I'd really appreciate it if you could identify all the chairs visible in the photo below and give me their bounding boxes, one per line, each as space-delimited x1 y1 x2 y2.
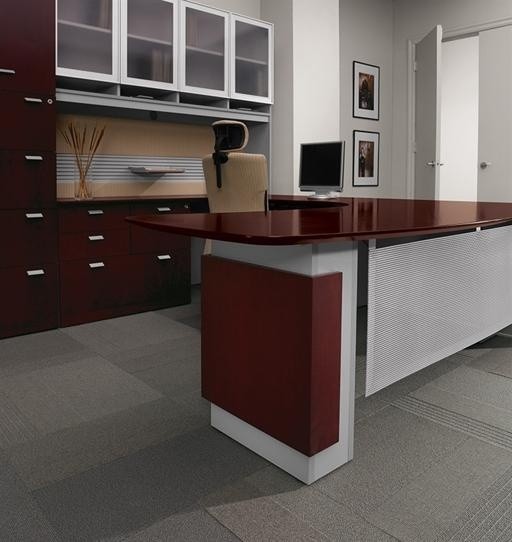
202 119 272 255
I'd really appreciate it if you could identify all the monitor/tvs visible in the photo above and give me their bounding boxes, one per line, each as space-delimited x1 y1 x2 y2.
299 140 346 199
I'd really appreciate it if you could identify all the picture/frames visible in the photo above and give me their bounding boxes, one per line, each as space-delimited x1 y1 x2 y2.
352 130 379 186
352 60 380 120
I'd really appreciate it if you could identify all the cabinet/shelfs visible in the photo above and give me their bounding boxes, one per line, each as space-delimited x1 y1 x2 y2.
0 0 58 338
177 0 275 105
55 0 177 90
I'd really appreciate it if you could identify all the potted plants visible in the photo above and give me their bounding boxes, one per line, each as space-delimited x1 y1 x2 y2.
56 120 105 200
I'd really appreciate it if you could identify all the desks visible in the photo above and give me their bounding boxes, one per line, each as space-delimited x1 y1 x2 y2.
59 191 512 487
